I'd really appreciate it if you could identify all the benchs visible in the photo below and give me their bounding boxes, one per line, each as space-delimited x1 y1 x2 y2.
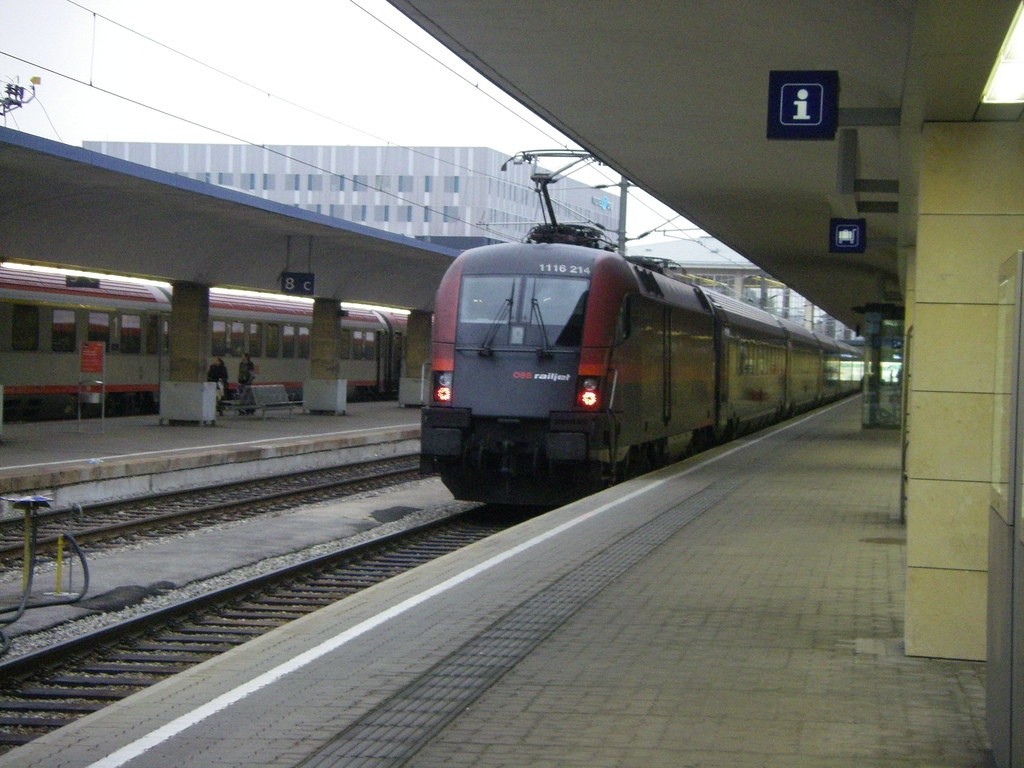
220 385 258 419
250 385 308 423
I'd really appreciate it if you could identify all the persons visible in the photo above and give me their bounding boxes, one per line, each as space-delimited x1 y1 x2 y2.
238 350 257 415
208 358 233 418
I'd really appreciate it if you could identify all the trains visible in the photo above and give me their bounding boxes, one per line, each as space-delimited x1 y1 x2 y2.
1 267 412 427
419 220 902 507
